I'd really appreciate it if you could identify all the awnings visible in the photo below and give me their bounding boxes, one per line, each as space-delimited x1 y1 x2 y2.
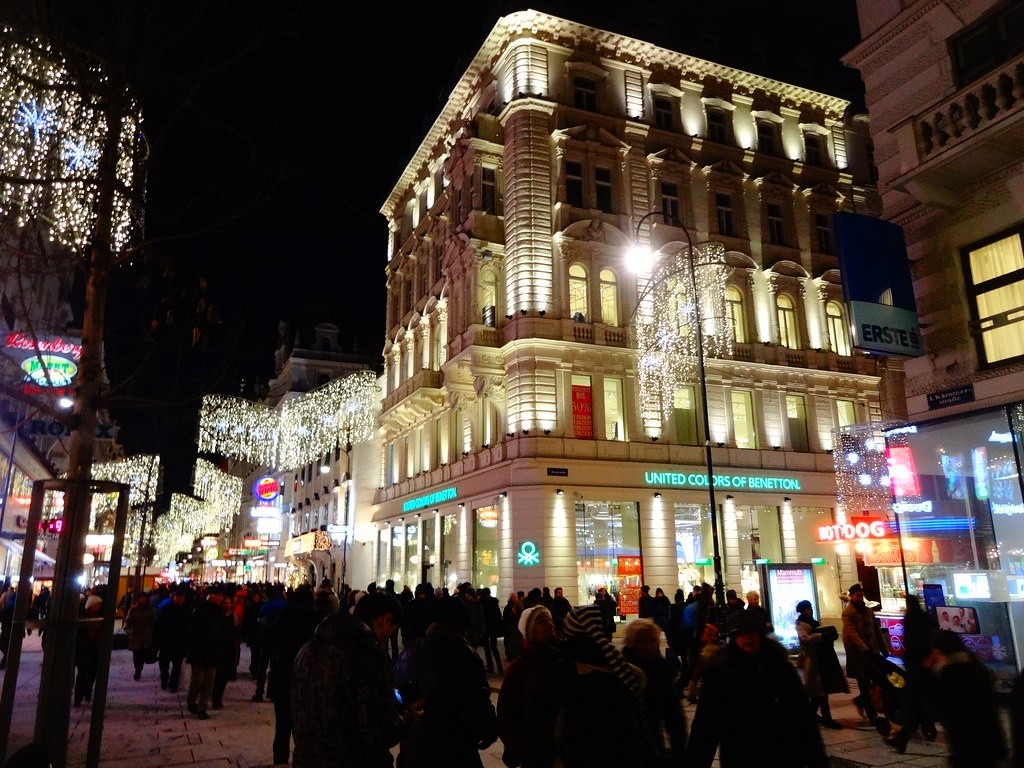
1 538 57 569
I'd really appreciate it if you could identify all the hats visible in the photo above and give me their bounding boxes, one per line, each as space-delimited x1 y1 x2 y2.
562 606 658 712
624 619 659 649
796 600 811 612
849 584 862 595
518 604 552 641
725 609 773 636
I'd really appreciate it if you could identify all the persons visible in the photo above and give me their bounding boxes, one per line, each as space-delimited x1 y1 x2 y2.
637 584 772 703
495 588 688 768
941 608 976 633
0 584 50 670
843 584 933 754
74 582 106 707
273 580 503 768
117 579 285 720
935 629 1024 768
687 616 827 768
796 600 850 728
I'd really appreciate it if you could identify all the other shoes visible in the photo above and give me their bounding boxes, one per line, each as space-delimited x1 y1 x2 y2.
187 703 198 714
484 663 494 672
823 721 842 729
86 695 92 701
854 698 868 719
197 710 208 720
883 737 906 754
213 701 223 710
167 683 177 693
161 679 168 690
687 695 699 704
74 702 81 707
249 695 263 702
134 671 141 681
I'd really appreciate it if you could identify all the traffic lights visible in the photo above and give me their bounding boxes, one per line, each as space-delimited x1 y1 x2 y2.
48 518 62 534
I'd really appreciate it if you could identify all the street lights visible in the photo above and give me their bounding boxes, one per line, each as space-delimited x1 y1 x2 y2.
319 445 351 590
624 211 725 604
174 520 243 586
1 364 76 540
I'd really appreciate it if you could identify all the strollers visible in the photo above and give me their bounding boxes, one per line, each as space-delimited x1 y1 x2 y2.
859 652 939 746
